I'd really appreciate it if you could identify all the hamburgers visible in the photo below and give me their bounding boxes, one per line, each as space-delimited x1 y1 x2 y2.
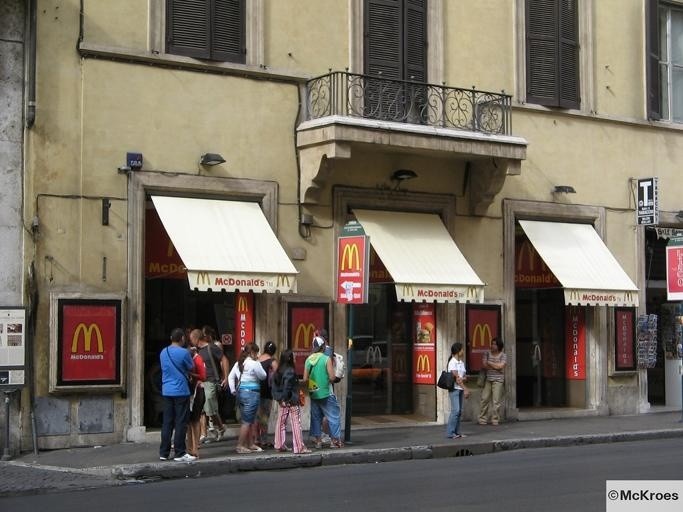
419 329 430 342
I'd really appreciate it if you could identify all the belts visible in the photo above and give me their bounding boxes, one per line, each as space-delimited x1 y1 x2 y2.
238 387 262 394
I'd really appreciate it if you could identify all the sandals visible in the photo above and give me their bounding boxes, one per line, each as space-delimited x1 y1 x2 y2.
234 445 252 454
248 445 264 452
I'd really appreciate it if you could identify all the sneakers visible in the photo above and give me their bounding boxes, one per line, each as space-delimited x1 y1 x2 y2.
255 440 273 449
303 448 312 454
276 445 292 452
198 415 226 446
173 452 199 462
160 453 172 461
312 432 345 449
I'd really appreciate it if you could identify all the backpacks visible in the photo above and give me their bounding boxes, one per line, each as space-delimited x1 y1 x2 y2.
332 352 346 383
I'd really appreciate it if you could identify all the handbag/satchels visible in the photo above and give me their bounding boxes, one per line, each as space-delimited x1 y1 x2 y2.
188 373 196 395
234 396 241 422
437 371 455 390
298 389 304 406
216 377 226 393
476 367 487 388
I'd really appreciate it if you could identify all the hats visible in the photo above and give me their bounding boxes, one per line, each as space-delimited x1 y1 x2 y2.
311 335 325 352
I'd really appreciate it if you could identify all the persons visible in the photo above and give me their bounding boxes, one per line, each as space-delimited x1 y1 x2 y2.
270 349 312 454
301 336 345 449
253 339 277 450
227 341 268 455
202 325 224 433
444 342 469 439
157 328 197 464
475 336 508 425
189 330 230 448
184 344 207 461
312 416 331 449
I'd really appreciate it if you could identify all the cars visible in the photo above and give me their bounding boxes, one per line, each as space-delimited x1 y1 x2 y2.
351 358 387 384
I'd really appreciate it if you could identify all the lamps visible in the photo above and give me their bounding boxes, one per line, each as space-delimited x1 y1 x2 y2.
392 168 419 181
200 153 226 167
553 185 576 194
